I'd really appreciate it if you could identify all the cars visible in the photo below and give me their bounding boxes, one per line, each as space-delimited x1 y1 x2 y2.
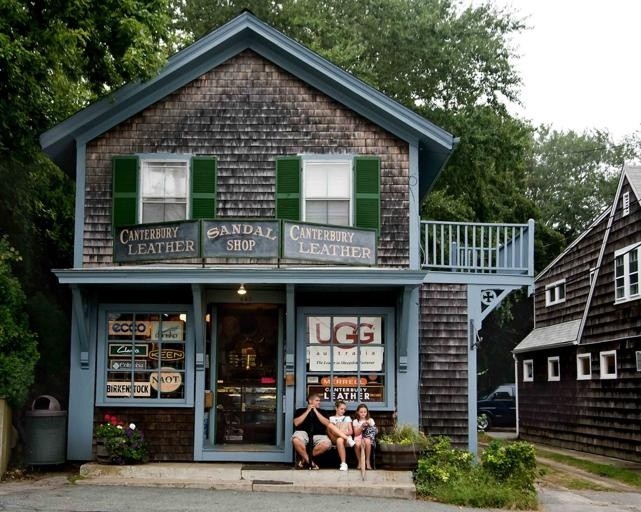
242 412 278 439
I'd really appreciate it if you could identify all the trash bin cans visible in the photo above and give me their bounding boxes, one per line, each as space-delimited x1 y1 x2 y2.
24 395 67 471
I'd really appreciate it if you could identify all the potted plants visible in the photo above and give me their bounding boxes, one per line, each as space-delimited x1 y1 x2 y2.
375 421 430 472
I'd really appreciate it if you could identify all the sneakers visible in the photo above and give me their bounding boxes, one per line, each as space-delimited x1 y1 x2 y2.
339 463 348 471
346 437 356 447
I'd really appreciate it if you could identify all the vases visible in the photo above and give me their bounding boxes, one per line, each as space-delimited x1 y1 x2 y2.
96 437 112 464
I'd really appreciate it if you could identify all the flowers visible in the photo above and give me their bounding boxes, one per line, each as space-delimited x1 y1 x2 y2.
94 412 151 466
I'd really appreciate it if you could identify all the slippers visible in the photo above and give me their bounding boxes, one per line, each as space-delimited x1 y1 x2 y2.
356 464 373 470
295 460 319 471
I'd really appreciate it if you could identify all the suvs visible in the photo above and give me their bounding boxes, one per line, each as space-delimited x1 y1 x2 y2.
477 383 517 432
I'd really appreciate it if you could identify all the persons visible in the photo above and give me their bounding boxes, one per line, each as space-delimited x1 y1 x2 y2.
353 403 377 470
326 401 355 471
292 394 332 469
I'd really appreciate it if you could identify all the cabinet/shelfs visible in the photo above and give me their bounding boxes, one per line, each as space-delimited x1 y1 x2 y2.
217 380 277 443
